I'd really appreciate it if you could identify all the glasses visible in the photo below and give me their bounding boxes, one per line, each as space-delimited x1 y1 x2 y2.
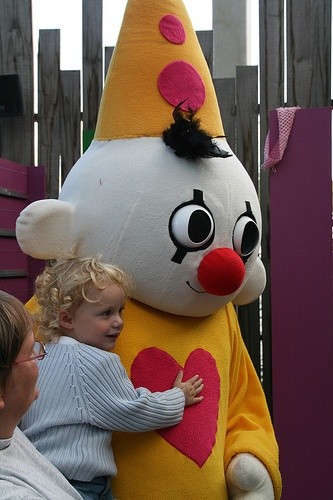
11 340 48 366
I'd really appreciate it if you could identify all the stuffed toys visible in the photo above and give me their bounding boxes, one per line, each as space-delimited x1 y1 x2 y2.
16 0 281 500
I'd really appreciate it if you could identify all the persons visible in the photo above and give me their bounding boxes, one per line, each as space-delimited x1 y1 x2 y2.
0 290 84 500
16 252 204 500
